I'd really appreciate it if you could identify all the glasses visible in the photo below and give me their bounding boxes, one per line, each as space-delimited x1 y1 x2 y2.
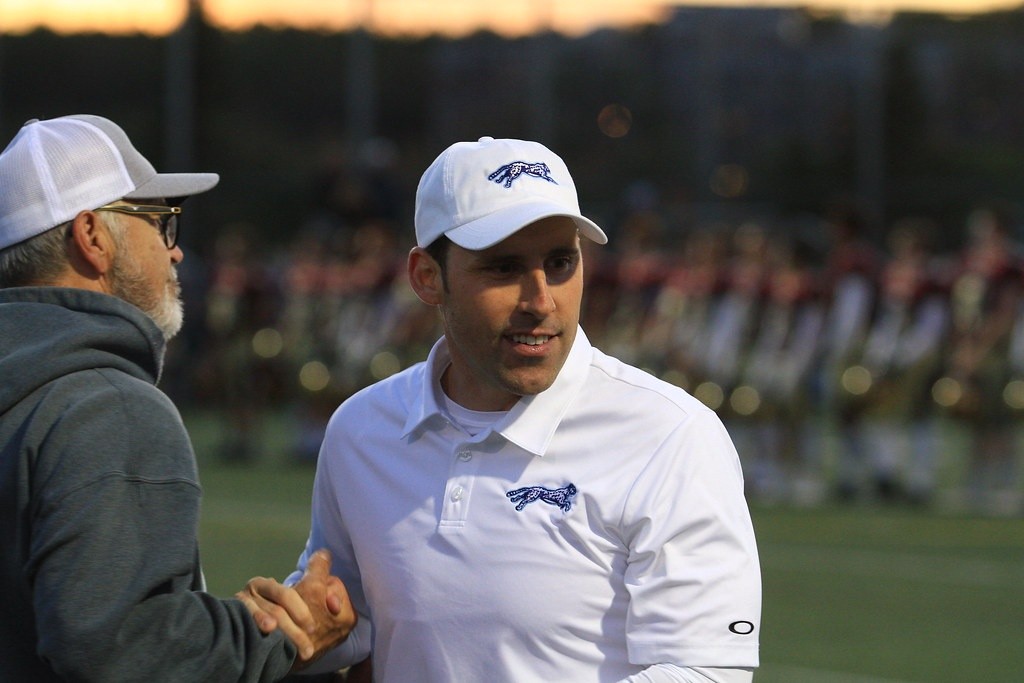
93 204 180 250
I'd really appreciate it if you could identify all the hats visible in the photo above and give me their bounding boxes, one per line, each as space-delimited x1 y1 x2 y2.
414 136 608 251
0 115 219 250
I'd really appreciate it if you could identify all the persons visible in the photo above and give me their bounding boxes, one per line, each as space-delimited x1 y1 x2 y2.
209 208 1024 511
232 135 764 683
0 110 356 682
